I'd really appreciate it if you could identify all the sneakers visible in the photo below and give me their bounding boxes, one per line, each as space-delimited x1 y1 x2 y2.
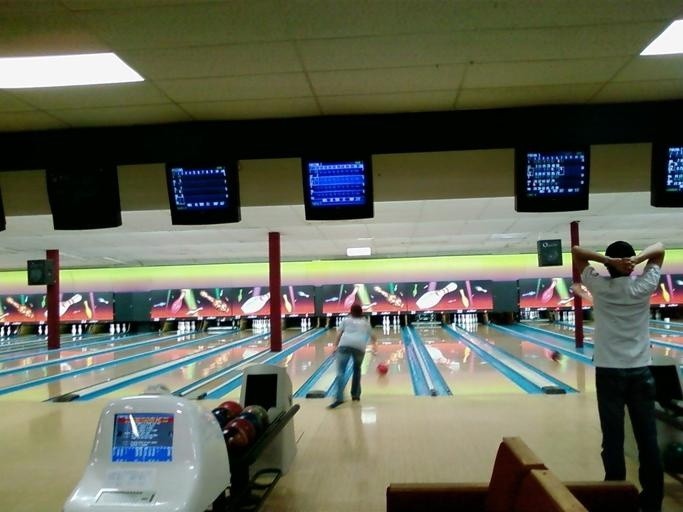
324 396 362 409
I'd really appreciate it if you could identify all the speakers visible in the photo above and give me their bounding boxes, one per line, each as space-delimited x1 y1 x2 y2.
27 260 54 285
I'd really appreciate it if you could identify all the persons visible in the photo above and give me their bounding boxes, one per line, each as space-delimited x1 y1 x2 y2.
567 239 667 512
325 304 379 410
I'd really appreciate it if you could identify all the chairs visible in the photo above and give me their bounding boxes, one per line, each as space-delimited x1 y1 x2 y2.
386 439 640 512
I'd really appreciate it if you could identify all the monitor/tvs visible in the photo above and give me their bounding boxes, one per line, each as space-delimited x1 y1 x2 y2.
245 373 276 408
647 365 682 399
302 151 375 221
43 162 124 231
514 141 591 213
112 412 172 465
165 161 242 225
650 137 683 208
